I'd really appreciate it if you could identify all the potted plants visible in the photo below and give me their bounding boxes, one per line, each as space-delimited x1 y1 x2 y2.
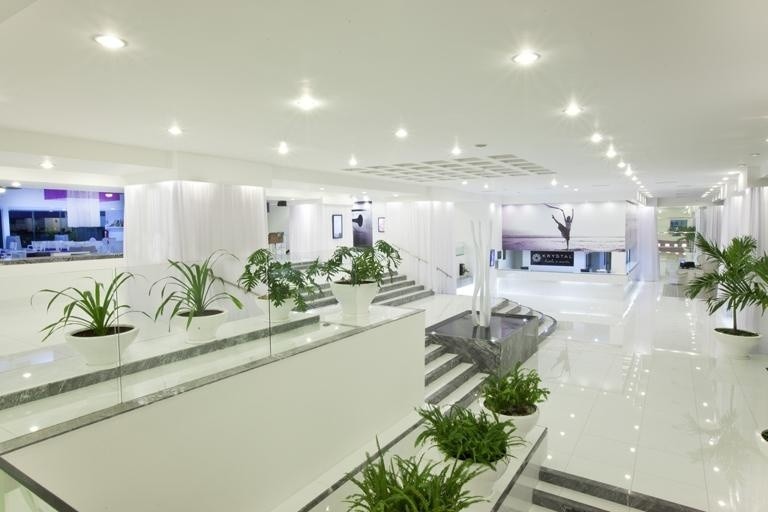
338 434 493 511
30 272 157 366
149 248 246 344
411 402 528 499
684 232 768 359
477 361 552 448
236 248 325 323
319 239 403 318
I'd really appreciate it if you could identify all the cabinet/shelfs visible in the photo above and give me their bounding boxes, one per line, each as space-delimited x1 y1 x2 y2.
383 399 551 512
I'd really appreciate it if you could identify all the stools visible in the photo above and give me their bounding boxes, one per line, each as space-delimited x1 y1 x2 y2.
665 263 689 285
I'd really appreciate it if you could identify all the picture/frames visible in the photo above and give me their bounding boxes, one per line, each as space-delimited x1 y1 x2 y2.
332 214 343 238
498 251 501 259
490 249 495 267
378 217 384 232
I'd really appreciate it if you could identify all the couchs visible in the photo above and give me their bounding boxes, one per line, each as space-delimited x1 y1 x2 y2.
696 252 721 285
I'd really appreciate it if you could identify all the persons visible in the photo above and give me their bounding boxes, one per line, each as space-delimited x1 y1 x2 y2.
552 208 574 249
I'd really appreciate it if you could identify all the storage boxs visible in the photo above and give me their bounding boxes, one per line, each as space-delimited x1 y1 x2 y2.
268 233 286 256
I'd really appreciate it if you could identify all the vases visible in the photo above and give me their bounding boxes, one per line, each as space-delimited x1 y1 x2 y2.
758 428 768 446
